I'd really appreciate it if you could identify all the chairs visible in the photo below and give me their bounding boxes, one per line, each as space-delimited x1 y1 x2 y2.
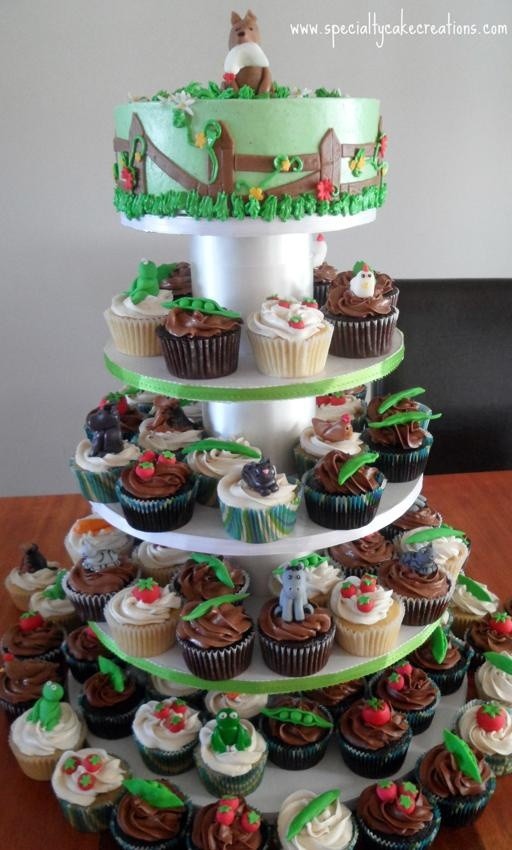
363 273 509 476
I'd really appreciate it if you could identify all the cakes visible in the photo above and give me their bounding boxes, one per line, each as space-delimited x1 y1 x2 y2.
0 9 512 850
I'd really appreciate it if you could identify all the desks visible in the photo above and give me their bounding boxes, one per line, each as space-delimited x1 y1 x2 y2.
1 463 511 847
